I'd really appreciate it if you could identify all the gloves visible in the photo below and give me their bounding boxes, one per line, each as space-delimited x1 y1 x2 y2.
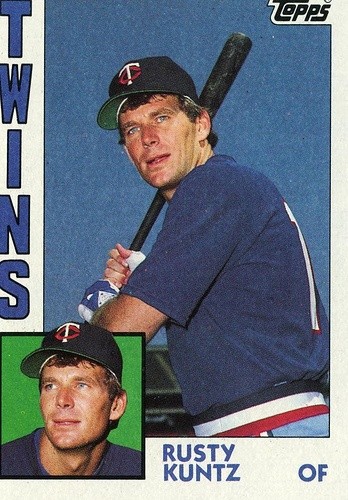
78 279 121 324
125 250 146 273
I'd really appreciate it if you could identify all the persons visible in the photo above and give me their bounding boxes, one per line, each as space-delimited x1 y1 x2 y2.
0 320 142 476
77 56 329 438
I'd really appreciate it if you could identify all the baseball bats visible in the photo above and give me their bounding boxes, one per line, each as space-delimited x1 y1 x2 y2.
129 32 253 252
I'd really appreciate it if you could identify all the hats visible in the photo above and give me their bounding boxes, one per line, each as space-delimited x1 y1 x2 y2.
19 321 123 385
97 55 200 131
